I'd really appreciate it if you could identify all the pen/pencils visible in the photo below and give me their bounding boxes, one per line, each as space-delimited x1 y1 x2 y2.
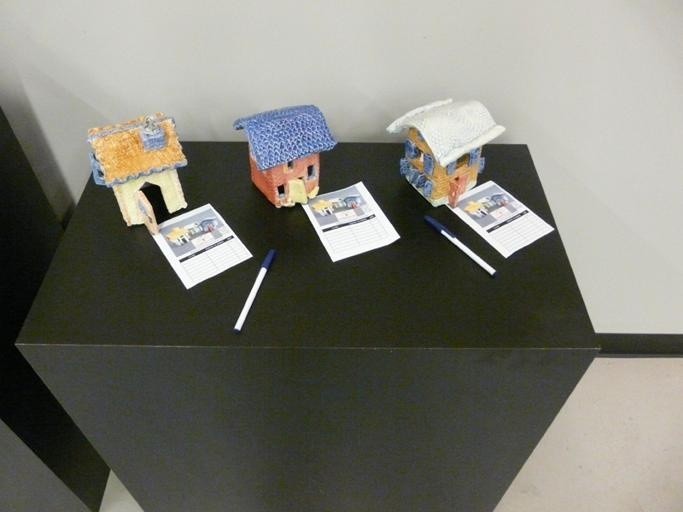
234 249 275 334
424 216 497 277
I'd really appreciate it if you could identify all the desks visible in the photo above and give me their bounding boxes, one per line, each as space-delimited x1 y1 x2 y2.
15 141 602 512
